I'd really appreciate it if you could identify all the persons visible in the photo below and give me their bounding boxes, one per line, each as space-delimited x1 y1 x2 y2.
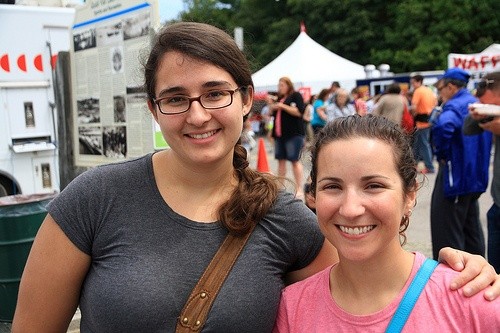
8 20 500 333
243 74 441 200
462 71 500 275
270 113 500 333
428 68 494 263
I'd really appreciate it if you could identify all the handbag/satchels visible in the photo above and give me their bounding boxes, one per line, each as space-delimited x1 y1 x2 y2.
402 111 414 134
415 113 430 123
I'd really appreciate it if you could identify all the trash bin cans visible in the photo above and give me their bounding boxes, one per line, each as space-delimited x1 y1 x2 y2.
0 190 58 323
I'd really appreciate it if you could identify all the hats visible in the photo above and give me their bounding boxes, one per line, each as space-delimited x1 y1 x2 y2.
434 67 472 88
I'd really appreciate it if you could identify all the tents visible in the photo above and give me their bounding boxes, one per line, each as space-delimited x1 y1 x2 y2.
251 22 394 96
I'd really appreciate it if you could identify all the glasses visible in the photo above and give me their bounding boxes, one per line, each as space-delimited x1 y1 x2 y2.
152 85 244 116
480 79 495 89
437 84 446 91
